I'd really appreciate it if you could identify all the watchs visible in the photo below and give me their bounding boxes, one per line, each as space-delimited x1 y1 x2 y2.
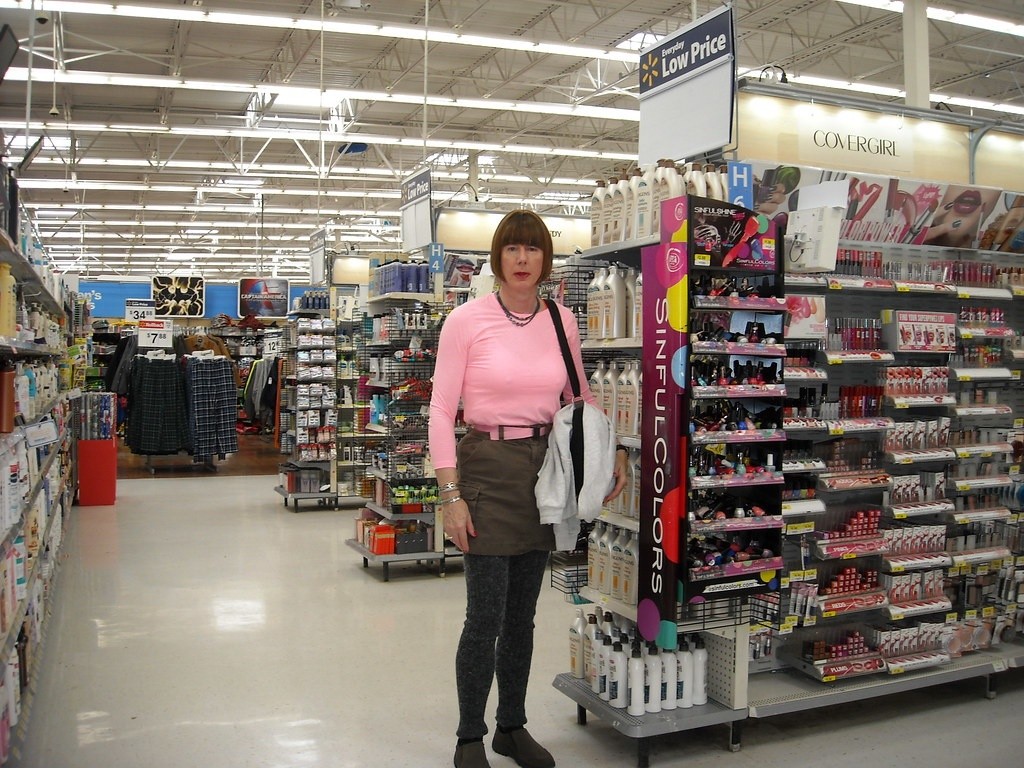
616 444 630 460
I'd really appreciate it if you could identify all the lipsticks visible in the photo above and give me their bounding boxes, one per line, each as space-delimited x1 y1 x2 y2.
840 176 1022 252
802 247 1024 662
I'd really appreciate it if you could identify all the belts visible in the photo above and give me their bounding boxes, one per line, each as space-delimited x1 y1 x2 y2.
473 424 546 441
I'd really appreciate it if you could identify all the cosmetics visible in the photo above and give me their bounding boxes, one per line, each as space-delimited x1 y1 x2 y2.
689 273 830 685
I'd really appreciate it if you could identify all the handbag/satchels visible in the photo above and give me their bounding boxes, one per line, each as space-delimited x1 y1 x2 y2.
569 406 586 497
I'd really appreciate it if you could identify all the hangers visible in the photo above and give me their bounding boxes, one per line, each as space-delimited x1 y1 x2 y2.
135 317 232 371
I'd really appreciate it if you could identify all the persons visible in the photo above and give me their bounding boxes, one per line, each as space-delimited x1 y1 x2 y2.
428 209 630 768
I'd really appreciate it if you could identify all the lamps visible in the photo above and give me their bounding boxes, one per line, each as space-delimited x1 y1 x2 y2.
0 1 910 277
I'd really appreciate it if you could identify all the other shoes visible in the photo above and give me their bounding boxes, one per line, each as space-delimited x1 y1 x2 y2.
491 727 556 768
454 742 491 768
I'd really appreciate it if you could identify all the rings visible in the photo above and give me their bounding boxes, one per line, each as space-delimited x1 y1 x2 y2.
446 536 453 541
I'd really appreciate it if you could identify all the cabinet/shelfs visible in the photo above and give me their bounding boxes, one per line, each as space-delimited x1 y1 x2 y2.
555 160 1024 765
209 311 283 444
1 151 96 765
346 241 613 578
88 320 135 437
272 275 375 513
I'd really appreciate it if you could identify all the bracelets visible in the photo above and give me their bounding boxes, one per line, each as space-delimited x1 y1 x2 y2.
440 495 460 507
437 481 460 495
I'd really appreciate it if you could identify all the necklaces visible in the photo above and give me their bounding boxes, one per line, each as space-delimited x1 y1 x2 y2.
496 290 541 327
